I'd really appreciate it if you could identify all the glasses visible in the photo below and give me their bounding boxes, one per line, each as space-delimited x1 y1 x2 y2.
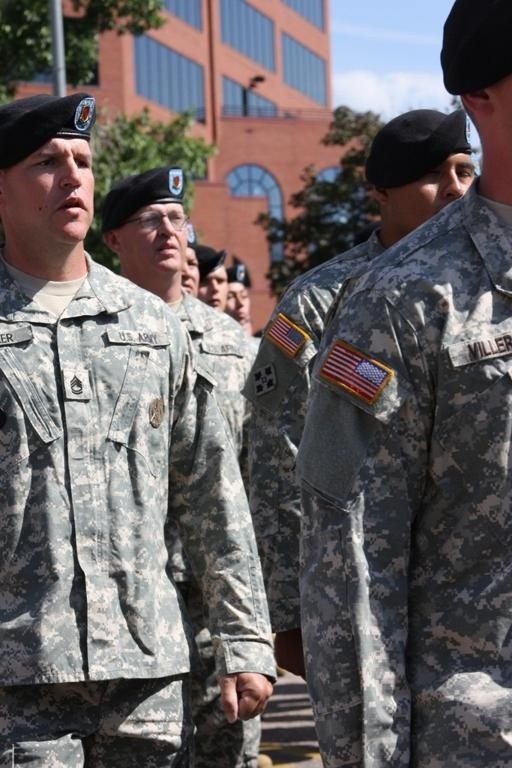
124 211 189 229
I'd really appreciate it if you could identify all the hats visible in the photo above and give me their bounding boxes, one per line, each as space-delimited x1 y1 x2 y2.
102 167 186 231
441 0 512 95
0 92 95 168
365 109 472 186
197 245 250 286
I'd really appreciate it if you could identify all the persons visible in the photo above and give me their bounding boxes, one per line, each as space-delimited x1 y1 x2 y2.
0 89 286 768
94 166 274 768
246 109 479 684
297 0 512 768
